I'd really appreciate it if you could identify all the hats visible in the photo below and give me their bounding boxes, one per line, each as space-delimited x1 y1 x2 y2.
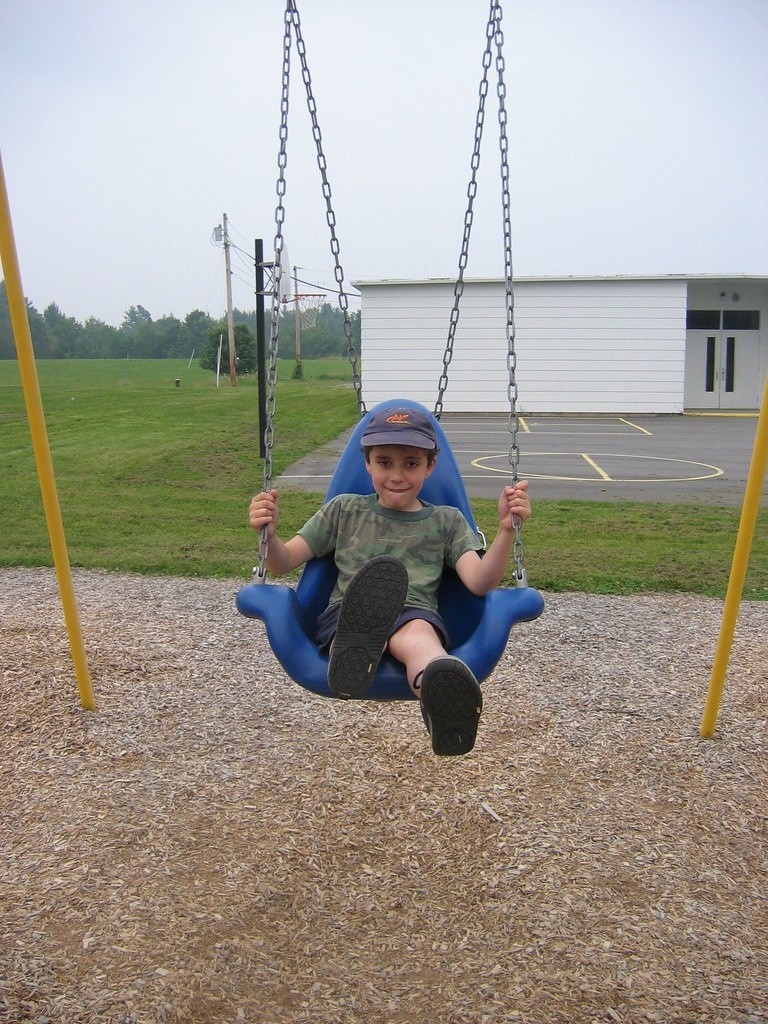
360 407 440 450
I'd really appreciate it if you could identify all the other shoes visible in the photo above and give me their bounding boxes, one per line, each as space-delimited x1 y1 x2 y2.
327 556 408 702
413 655 483 756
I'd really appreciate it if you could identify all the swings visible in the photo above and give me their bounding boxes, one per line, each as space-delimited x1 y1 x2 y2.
232 0 548 705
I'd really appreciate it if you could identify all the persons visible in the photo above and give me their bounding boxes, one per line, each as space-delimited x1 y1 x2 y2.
250 407 532 756
